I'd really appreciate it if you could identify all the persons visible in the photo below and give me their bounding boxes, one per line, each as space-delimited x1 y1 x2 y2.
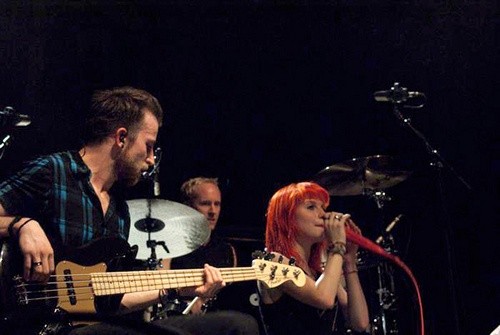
256 182 370 335
156 177 237 270
0 87 261 335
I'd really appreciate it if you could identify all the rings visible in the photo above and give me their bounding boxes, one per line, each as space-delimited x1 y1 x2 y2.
33 261 42 266
335 216 340 219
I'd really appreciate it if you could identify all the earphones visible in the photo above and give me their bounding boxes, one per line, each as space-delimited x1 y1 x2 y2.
120 132 124 143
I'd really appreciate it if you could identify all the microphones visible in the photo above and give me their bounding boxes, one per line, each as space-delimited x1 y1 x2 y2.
344 227 393 258
373 82 428 103
374 214 402 245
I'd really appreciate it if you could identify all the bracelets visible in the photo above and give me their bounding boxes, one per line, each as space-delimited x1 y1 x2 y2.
159 287 180 303
326 240 348 257
8 216 33 238
344 270 359 275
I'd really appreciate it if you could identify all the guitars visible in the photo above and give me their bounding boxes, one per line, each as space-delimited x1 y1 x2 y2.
0 231 308 335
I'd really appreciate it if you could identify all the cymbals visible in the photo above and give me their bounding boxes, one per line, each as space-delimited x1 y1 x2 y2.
310 154 417 196
123 199 211 260
